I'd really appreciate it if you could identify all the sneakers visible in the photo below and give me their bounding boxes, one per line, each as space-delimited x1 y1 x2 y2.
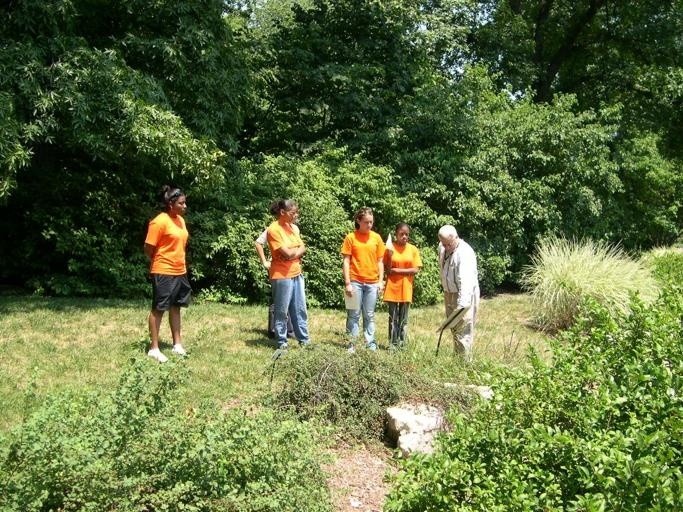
148 349 168 363
173 344 184 353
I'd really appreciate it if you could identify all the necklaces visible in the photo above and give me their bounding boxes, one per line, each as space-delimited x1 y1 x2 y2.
443 238 461 262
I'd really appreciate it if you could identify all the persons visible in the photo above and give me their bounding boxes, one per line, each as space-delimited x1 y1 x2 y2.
383 220 423 346
266 199 314 349
144 186 190 364
254 199 308 340
340 205 383 351
435 226 481 366
